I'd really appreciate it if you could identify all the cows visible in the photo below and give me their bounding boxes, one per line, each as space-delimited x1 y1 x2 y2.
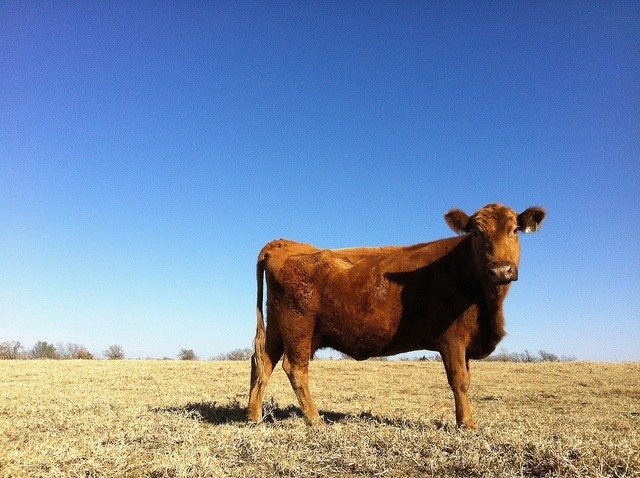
246 200 549 433
